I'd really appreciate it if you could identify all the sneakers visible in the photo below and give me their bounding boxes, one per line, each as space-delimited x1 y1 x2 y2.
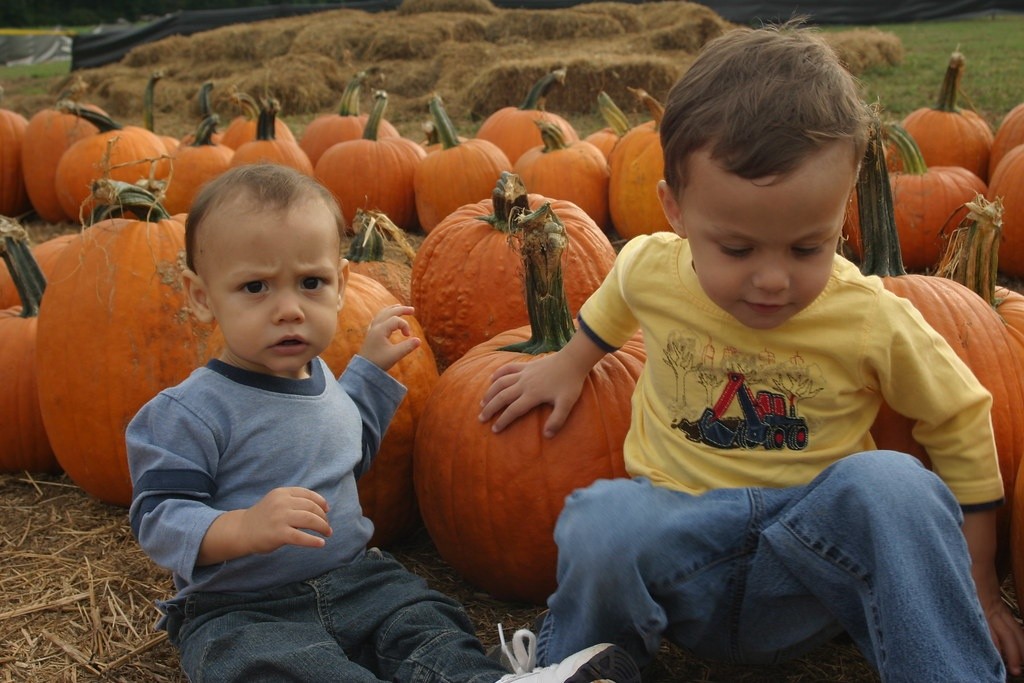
496 642 641 683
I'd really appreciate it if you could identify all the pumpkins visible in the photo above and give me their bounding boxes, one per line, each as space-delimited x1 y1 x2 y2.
836 50 1024 619
0 71 669 601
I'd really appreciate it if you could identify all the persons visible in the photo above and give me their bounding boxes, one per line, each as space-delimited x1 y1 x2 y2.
124 166 644 683
478 26 1024 683
